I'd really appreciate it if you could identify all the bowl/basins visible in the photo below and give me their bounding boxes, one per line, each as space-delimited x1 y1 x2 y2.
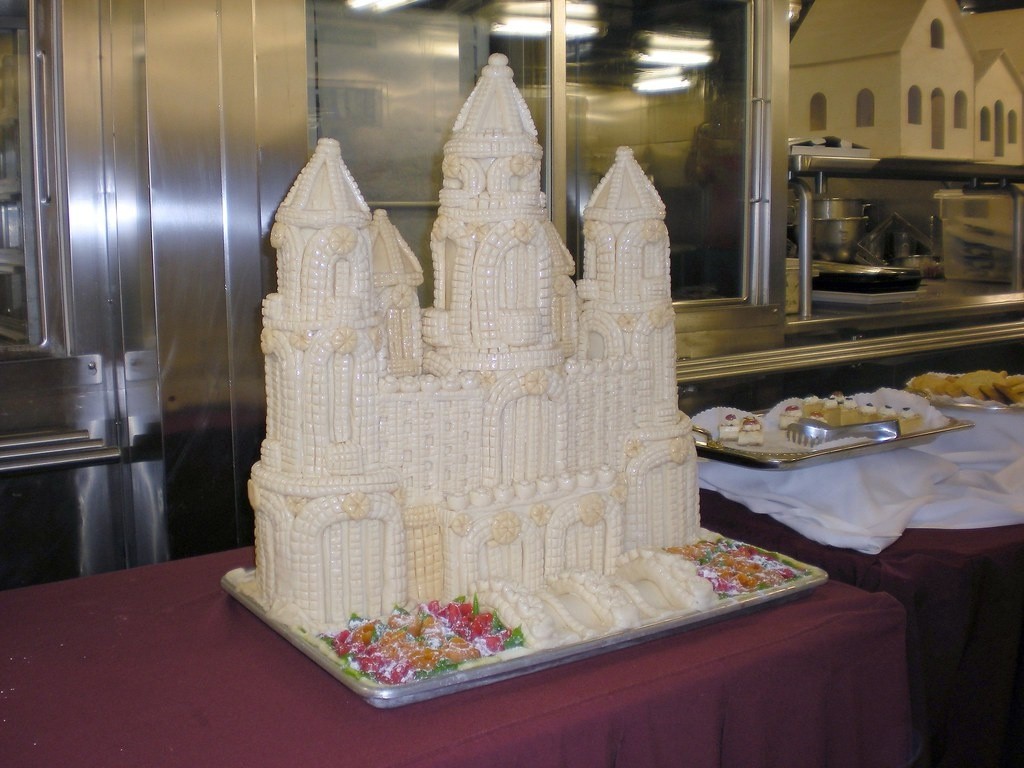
890 254 941 276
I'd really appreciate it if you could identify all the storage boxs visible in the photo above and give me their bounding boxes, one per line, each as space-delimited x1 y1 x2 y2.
933 188 1020 283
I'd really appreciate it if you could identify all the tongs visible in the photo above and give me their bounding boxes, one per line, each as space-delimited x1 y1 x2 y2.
786 417 900 448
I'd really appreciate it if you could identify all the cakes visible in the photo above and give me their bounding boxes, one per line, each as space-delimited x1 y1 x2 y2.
226 52 813 688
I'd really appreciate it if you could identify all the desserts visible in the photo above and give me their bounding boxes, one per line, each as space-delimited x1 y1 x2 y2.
718 396 922 447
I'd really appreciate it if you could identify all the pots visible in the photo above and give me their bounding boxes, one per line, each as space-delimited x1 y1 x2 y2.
812 198 876 261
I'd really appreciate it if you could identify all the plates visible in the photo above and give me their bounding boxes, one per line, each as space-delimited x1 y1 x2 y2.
811 270 927 305
914 393 1024 412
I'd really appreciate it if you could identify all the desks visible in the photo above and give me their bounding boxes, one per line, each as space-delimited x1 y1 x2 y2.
699 403 1024 768
0 542 910 767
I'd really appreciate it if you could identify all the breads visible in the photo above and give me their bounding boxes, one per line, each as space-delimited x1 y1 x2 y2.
909 368 1024 406
